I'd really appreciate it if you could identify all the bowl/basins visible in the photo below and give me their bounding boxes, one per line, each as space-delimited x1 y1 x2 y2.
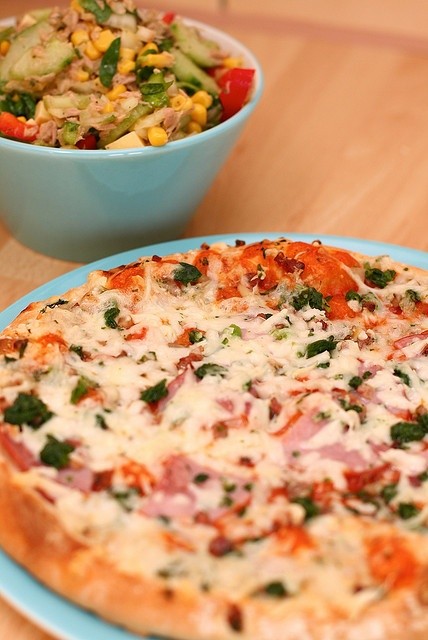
1 6 267 262
